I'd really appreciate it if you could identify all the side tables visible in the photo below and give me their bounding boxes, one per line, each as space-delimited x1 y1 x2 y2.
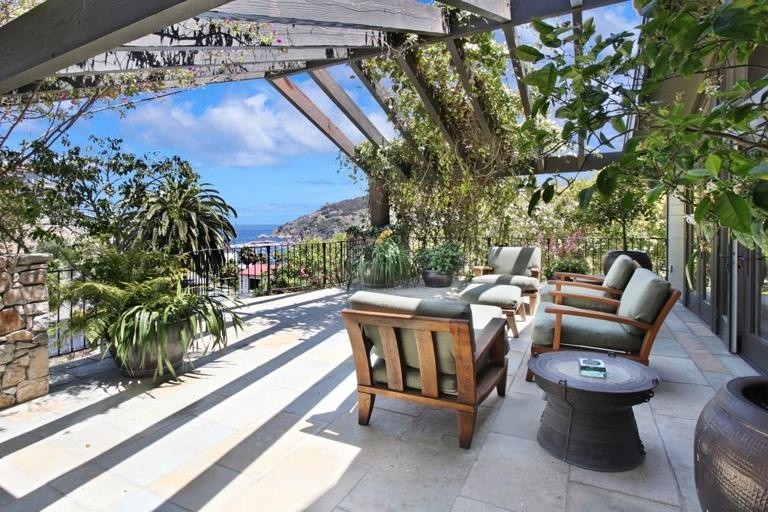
527 352 660 473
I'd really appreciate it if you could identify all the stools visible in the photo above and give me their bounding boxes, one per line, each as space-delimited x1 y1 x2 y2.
458 283 527 338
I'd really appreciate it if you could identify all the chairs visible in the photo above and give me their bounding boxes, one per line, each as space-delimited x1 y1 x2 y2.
471 247 542 317
340 291 509 450
527 267 683 382
536 254 644 314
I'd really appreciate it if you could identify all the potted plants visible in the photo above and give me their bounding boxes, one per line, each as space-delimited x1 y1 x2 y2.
410 241 463 288
345 236 418 289
512 0 766 508
39 232 249 385
